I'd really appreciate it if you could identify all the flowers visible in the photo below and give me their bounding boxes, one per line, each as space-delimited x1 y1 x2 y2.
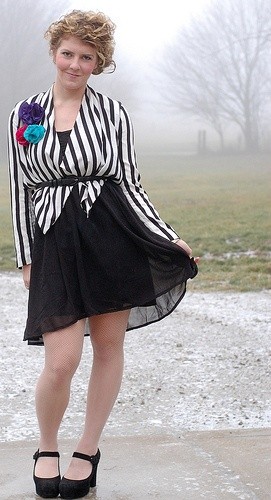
15 101 46 147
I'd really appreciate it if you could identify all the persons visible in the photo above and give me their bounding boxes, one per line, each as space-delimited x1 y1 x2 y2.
5 9 206 500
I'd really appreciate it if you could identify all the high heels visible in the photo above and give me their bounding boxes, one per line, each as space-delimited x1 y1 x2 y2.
59 449 100 499
31 449 61 498
